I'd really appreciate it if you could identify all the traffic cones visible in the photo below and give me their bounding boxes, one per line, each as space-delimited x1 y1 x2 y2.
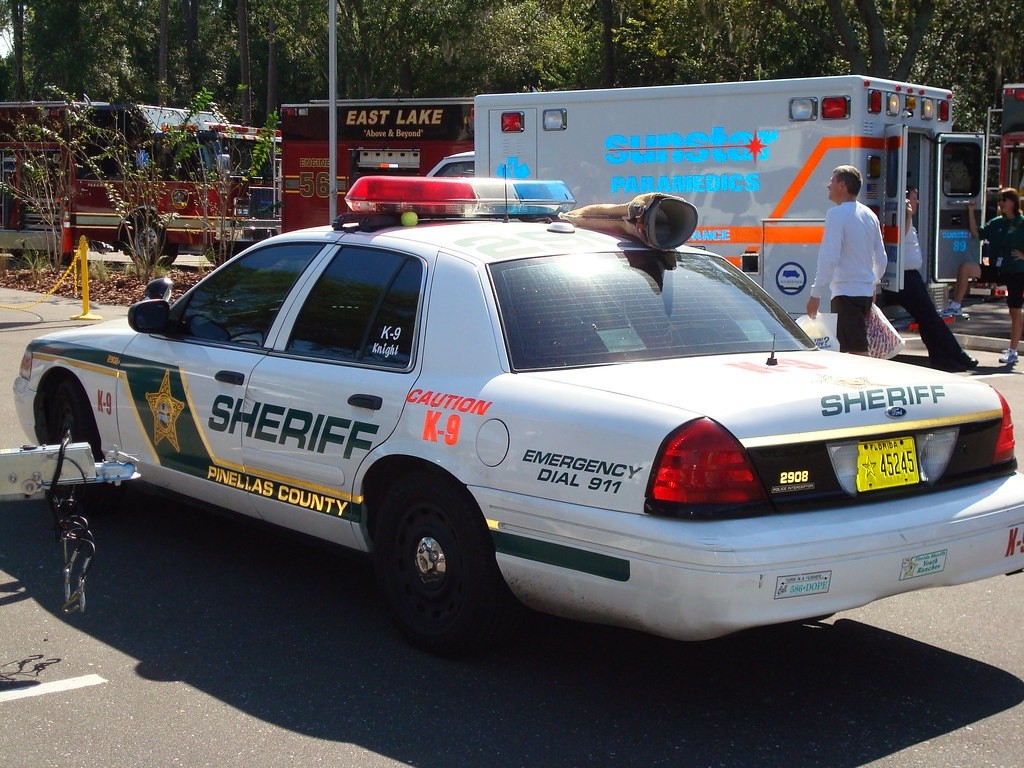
57 204 78 266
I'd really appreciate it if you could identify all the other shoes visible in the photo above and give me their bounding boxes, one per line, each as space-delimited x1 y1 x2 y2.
956 353 978 369
945 361 966 371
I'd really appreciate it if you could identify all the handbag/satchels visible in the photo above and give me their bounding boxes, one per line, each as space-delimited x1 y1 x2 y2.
863 302 905 360
795 312 840 352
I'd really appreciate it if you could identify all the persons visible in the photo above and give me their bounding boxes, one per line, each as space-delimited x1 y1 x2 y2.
891 185 977 372
805 163 888 357
939 186 1023 362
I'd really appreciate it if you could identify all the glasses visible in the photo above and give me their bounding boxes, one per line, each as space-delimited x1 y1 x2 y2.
998 198 1007 202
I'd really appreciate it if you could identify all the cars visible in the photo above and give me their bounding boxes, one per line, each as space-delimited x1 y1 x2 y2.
12 174 1024 660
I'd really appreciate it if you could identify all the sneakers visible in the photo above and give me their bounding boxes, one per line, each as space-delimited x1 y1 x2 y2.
937 301 962 317
999 348 1019 363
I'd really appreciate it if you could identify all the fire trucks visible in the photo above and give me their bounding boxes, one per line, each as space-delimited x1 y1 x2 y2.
1 102 285 268
420 75 988 339
999 84 1024 226
